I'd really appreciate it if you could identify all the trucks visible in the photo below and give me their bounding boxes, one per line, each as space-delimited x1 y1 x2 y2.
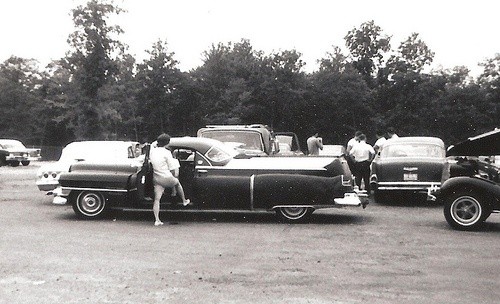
196 123 301 159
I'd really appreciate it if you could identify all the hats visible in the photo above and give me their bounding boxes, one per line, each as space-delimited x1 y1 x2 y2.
156 133 170 147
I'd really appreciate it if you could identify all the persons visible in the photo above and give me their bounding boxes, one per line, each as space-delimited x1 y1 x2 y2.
307 130 324 156
149 133 190 226
346 127 399 196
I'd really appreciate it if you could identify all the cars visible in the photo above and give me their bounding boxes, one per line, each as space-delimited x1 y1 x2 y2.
369 137 451 206
0 139 42 167
431 128 500 231
58 137 369 225
308 145 347 158
37 140 142 196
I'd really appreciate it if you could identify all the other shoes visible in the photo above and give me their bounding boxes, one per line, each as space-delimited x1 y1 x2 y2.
154 220 164 226
182 199 191 206
